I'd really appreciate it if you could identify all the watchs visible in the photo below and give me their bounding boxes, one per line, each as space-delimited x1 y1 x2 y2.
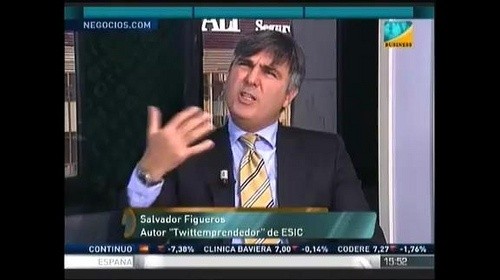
136 162 163 188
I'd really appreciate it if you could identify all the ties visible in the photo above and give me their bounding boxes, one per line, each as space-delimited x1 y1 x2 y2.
238 133 281 247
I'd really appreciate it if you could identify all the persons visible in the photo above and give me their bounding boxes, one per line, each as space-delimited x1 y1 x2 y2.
116 28 387 245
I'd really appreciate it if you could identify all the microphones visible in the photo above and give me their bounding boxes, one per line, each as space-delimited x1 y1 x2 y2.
216 167 230 185
